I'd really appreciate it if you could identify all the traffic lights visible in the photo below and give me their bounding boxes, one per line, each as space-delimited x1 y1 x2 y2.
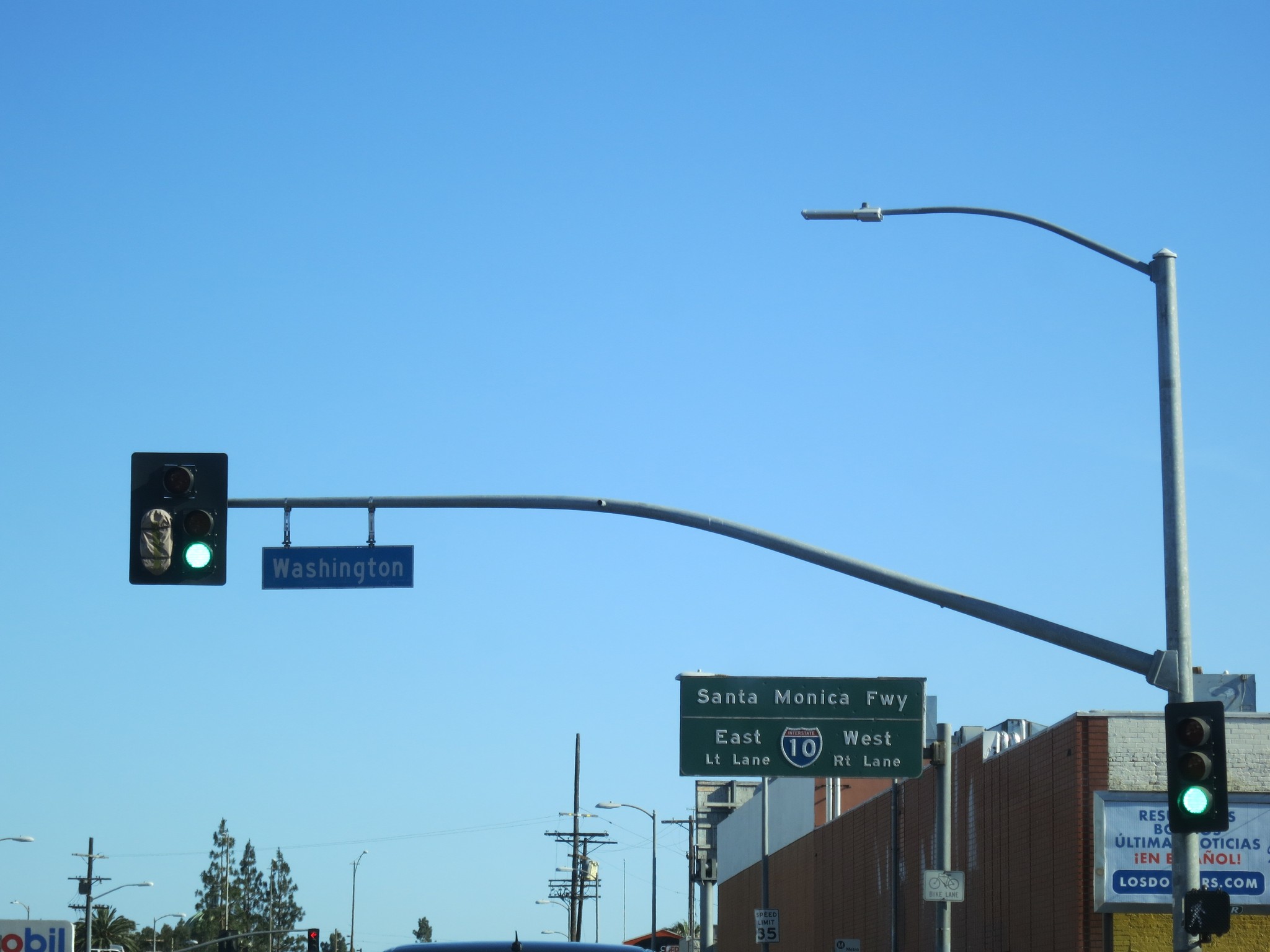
1184 884 1231 944
308 928 319 952
1164 701 1229 834
129 451 229 586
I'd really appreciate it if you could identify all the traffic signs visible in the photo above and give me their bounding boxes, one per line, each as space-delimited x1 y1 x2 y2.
754 908 780 944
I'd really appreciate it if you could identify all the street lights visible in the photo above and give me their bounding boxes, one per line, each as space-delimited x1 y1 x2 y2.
535 899 571 943
349 850 369 952
86 881 154 952
153 912 186 952
799 201 1202 952
556 867 599 943
595 801 657 951
10 900 30 920
